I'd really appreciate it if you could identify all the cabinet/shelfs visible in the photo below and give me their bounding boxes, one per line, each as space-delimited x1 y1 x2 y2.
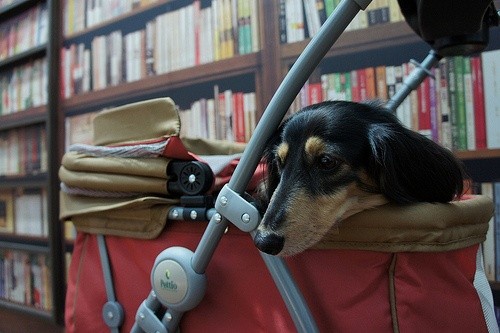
0 0 500 327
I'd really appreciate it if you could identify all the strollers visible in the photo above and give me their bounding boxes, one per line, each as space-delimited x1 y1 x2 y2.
57 0 499 333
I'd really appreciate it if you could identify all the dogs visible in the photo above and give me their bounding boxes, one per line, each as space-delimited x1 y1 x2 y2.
251 99 475 258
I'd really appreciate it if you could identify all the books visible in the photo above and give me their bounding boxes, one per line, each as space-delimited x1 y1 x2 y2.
0 0 499 313
